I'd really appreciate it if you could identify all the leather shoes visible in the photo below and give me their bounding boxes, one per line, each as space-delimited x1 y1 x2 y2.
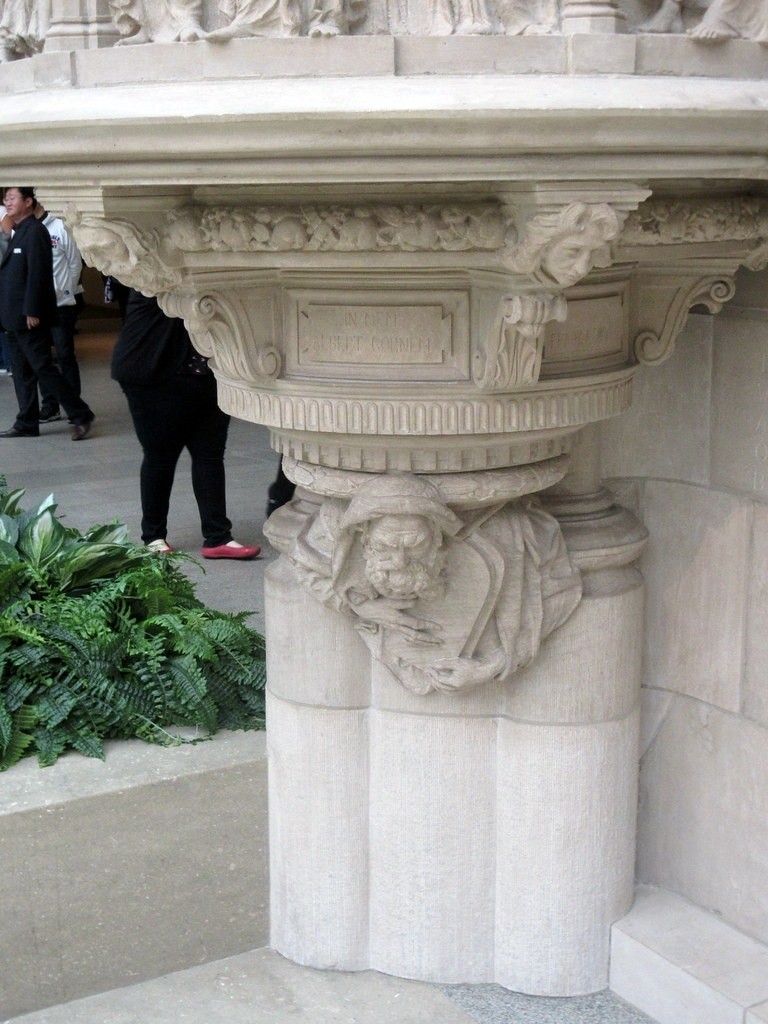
72 419 90 441
0 427 38 436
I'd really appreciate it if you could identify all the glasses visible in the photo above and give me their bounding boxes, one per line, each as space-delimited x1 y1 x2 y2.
3 196 24 204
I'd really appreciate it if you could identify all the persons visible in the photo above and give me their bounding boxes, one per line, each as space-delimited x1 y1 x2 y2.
0 186 95 442
109 281 261 570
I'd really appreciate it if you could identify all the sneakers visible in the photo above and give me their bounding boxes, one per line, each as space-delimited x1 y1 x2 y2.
38 404 61 423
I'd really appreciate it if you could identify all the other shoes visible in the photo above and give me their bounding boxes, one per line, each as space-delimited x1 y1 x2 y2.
200 543 261 558
160 541 174 553
